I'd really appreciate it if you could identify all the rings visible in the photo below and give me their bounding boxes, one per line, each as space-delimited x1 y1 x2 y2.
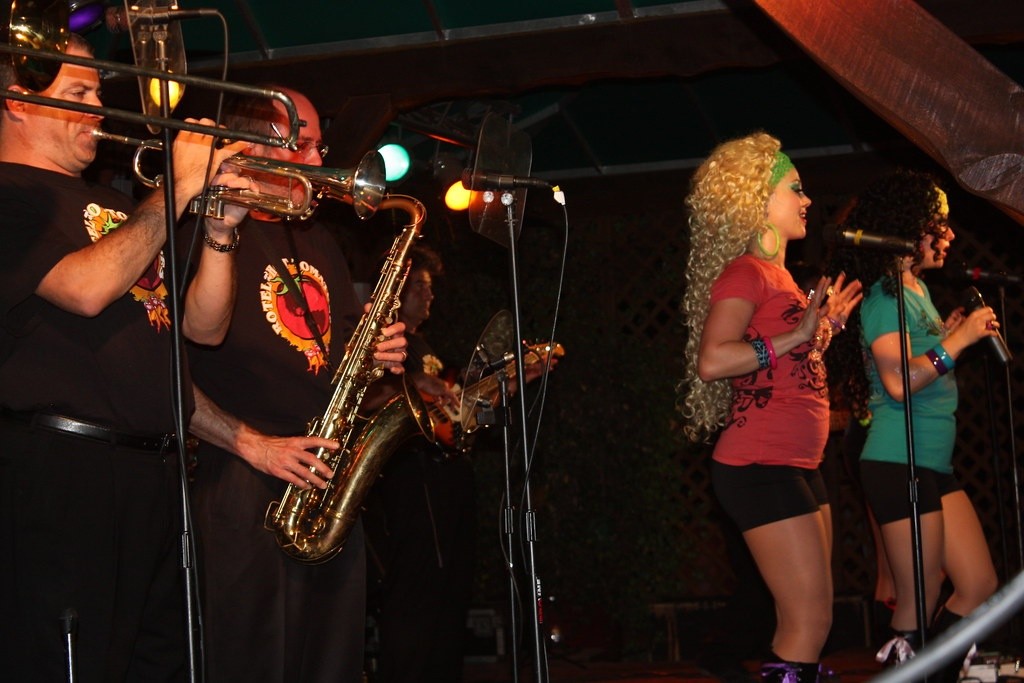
948 313 957 321
985 319 994 330
826 285 839 296
402 351 407 361
807 287 815 301
243 173 254 184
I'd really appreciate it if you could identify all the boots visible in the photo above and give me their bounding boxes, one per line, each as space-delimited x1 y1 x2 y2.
924 606 973 683
871 596 897 652
758 649 821 683
876 627 927 683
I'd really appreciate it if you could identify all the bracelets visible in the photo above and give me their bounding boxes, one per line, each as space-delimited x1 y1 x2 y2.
826 315 847 332
925 342 957 376
200 227 241 252
749 333 777 371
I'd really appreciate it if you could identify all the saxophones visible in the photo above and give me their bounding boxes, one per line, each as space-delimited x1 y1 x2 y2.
262 192 428 566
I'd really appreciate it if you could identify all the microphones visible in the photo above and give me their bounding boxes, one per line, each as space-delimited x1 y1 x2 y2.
961 285 1013 366
837 226 916 252
473 348 530 381
967 267 1024 285
463 167 553 191
106 7 218 35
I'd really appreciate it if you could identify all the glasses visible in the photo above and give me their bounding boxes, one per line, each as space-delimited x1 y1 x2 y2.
296 141 329 159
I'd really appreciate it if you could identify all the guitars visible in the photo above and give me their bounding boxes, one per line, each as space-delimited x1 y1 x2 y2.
402 336 565 455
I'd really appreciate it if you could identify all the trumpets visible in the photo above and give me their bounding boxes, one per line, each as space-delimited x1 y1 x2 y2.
0 0 300 156
87 121 387 224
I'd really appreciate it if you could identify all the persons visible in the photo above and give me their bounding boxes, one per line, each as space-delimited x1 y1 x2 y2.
857 172 1001 683
370 244 557 682
683 133 864 683
171 83 410 683
0 22 259 683
865 503 947 670
